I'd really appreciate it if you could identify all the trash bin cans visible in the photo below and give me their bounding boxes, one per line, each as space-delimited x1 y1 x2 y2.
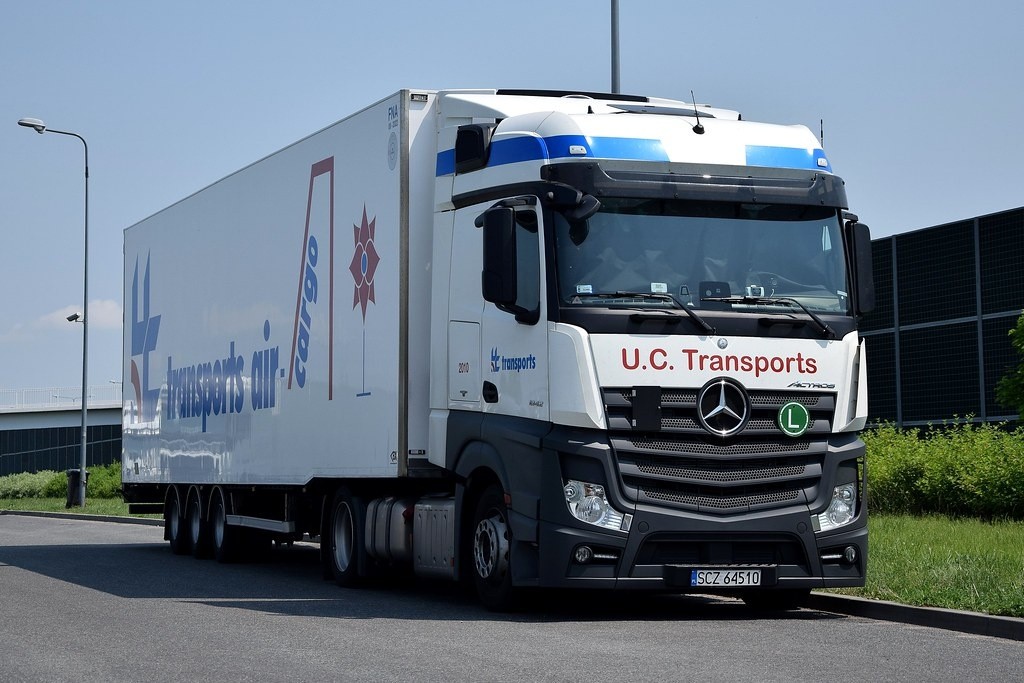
64 469 91 508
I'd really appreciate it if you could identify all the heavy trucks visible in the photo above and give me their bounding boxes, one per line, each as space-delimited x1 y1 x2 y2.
116 87 878 613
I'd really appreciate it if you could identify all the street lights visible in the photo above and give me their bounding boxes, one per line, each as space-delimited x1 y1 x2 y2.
109 379 122 386
52 395 95 405
17 117 88 509
